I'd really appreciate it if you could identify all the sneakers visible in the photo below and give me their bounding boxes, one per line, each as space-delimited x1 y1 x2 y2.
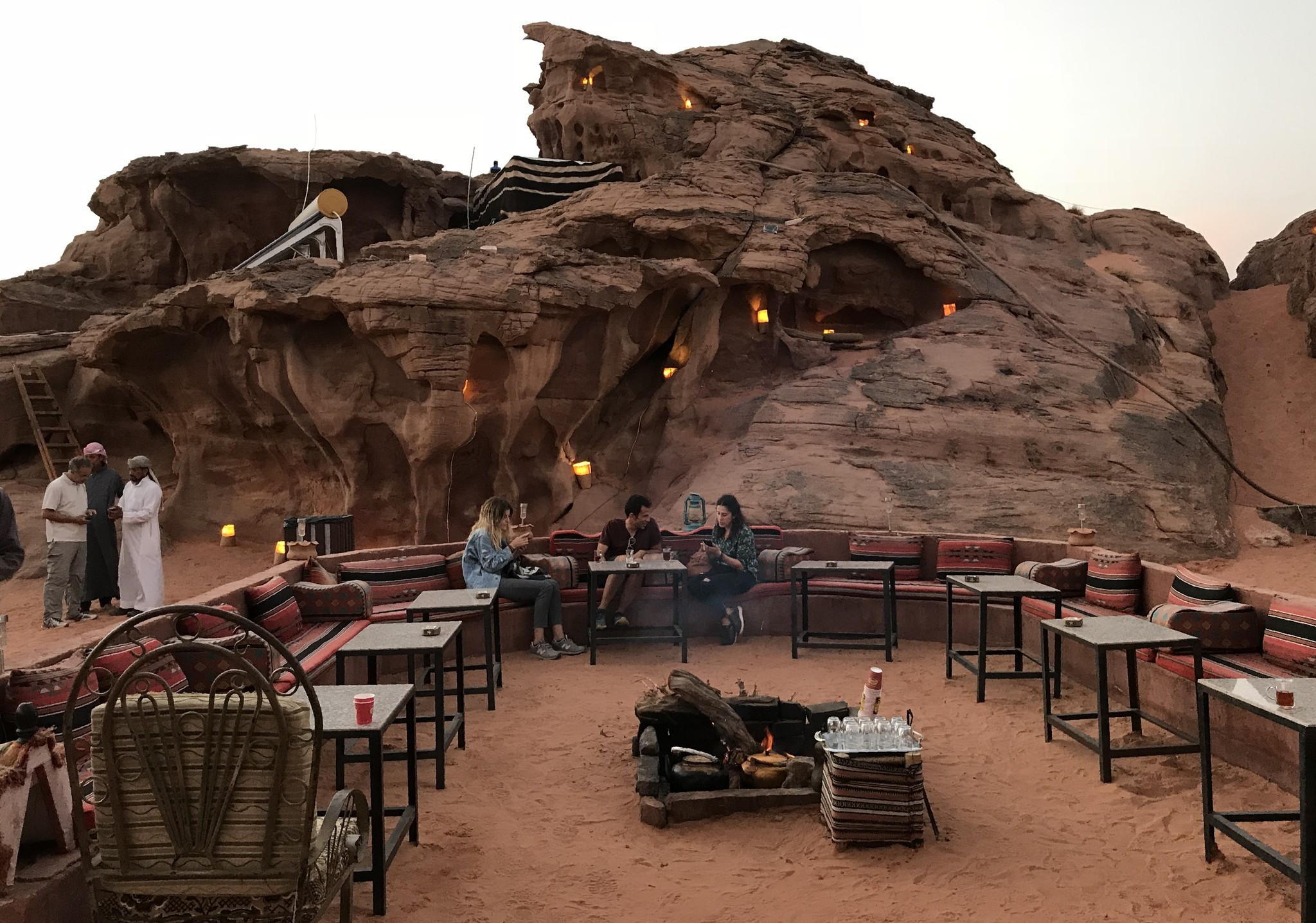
530 641 562 660
729 605 744 635
551 634 586 655
100 603 117 612
595 607 607 630
43 617 69 628
67 612 97 622
721 622 737 646
613 612 627 627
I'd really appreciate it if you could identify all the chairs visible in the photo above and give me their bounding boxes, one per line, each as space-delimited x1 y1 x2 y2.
62 603 373 923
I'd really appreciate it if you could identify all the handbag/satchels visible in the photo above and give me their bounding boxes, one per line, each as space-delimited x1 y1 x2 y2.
686 547 713 577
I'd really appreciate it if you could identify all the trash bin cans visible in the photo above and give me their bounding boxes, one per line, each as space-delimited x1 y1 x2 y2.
283 514 355 562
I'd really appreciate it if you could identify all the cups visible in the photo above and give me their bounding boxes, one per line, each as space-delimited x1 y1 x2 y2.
1266 677 1295 709
827 667 913 751
354 693 376 725
626 549 634 563
663 548 672 562
593 549 603 564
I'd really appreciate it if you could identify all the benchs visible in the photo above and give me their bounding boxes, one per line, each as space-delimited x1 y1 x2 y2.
0 526 1308 923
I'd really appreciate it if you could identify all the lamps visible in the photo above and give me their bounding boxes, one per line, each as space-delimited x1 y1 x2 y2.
221 524 235 537
685 99 691 109
572 461 591 475
275 541 285 554
757 309 769 323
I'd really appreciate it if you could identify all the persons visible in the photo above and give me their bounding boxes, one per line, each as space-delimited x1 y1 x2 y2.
688 494 760 646
106 455 166 616
592 493 665 630
77 442 127 614
488 160 501 173
461 495 589 660
40 456 97 628
0 485 27 588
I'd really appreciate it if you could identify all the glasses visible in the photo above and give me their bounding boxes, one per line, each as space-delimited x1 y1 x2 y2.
72 470 93 478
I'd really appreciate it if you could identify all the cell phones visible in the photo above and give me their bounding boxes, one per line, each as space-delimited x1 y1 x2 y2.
517 533 530 551
703 540 712 547
109 507 117 511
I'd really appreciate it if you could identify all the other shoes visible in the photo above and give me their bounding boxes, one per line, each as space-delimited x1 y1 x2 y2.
109 607 134 616
127 609 143 616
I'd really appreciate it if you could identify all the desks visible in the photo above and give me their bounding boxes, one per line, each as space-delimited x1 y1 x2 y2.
588 559 689 663
1038 614 1204 782
945 575 1062 705
407 587 503 711
1196 677 1316 923
790 560 898 662
284 682 418 916
335 619 463 791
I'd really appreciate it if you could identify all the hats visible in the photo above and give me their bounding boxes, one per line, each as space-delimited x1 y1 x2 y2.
127 456 164 511
84 443 109 466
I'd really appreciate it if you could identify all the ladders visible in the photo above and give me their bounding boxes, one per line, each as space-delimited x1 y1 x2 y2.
11 360 84 481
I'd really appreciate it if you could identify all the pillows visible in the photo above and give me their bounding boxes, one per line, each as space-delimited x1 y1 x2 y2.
83 635 191 705
847 529 926 581
1085 547 1141 616
1263 596 1316 678
444 549 468 590
245 573 303 643
176 603 245 639
3 648 104 765
301 554 338 588
933 536 1014 584
1166 564 1233 609
